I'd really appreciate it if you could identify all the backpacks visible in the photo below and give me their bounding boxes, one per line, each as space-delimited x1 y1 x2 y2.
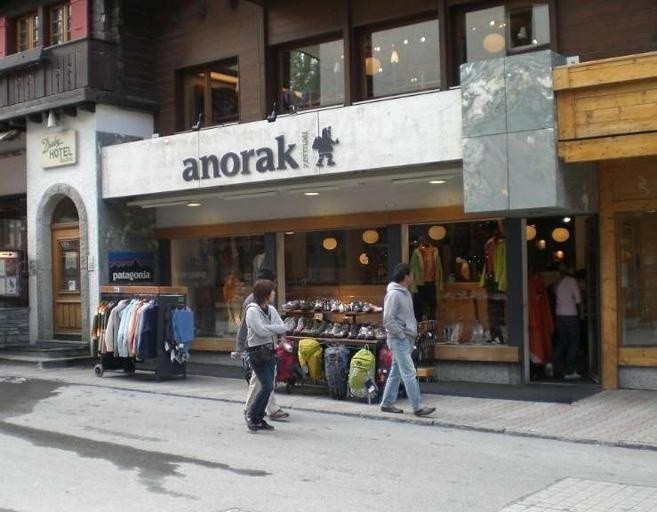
347 344 375 399
275 337 295 388
378 343 394 385
297 339 323 380
323 343 350 400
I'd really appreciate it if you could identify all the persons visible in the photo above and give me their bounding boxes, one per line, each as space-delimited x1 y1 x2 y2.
245 280 287 431
555 265 583 380
380 263 436 417
410 236 443 322
231 269 289 420
479 231 507 344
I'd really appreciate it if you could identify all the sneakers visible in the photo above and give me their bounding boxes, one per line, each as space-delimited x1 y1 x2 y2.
270 408 290 421
564 369 582 379
256 417 275 431
243 415 258 434
544 361 554 377
380 404 405 413
414 406 436 417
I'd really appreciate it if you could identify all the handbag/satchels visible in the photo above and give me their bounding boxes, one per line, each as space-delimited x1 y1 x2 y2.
253 345 275 364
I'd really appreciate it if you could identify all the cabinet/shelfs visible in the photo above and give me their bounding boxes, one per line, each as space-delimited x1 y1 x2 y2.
276 306 384 403
93 285 189 383
